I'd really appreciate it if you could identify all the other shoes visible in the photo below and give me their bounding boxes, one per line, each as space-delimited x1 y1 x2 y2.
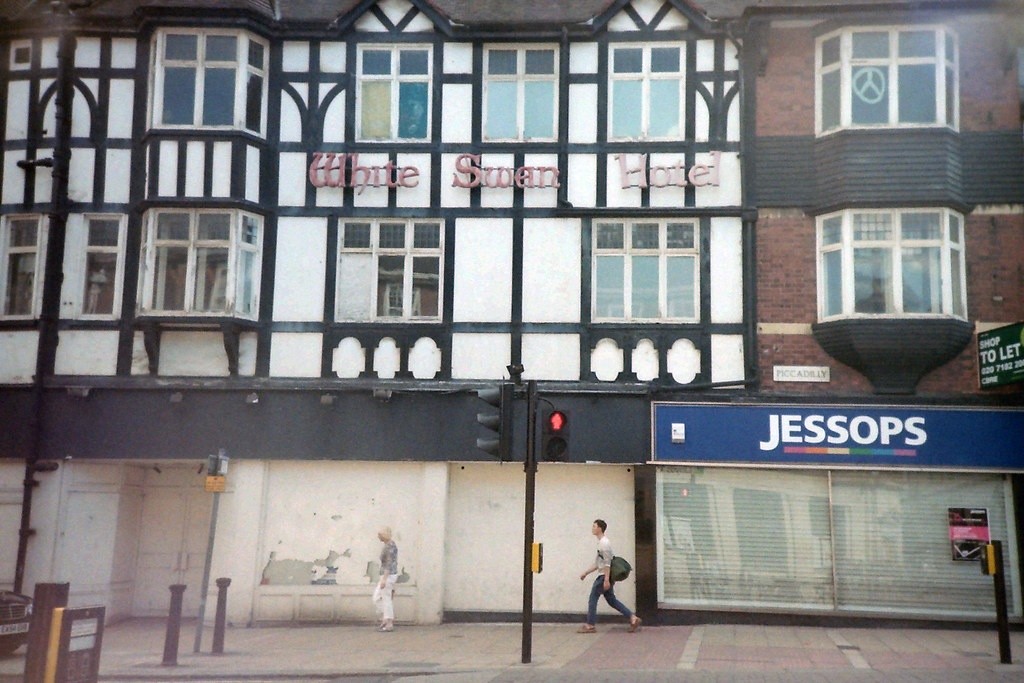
629 617 642 633
577 626 596 633
376 623 393 632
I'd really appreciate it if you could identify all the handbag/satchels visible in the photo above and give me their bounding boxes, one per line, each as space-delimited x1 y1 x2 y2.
610 556 631 581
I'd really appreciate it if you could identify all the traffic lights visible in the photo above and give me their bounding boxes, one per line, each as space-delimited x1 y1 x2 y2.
541 409 570 461
477 381 515 462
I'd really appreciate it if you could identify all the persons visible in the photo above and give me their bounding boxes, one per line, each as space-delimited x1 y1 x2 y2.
373 527 398 632
87 269 106 313
577 519 642 633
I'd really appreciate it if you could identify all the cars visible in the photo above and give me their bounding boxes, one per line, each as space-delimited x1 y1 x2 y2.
0 589 33 654
